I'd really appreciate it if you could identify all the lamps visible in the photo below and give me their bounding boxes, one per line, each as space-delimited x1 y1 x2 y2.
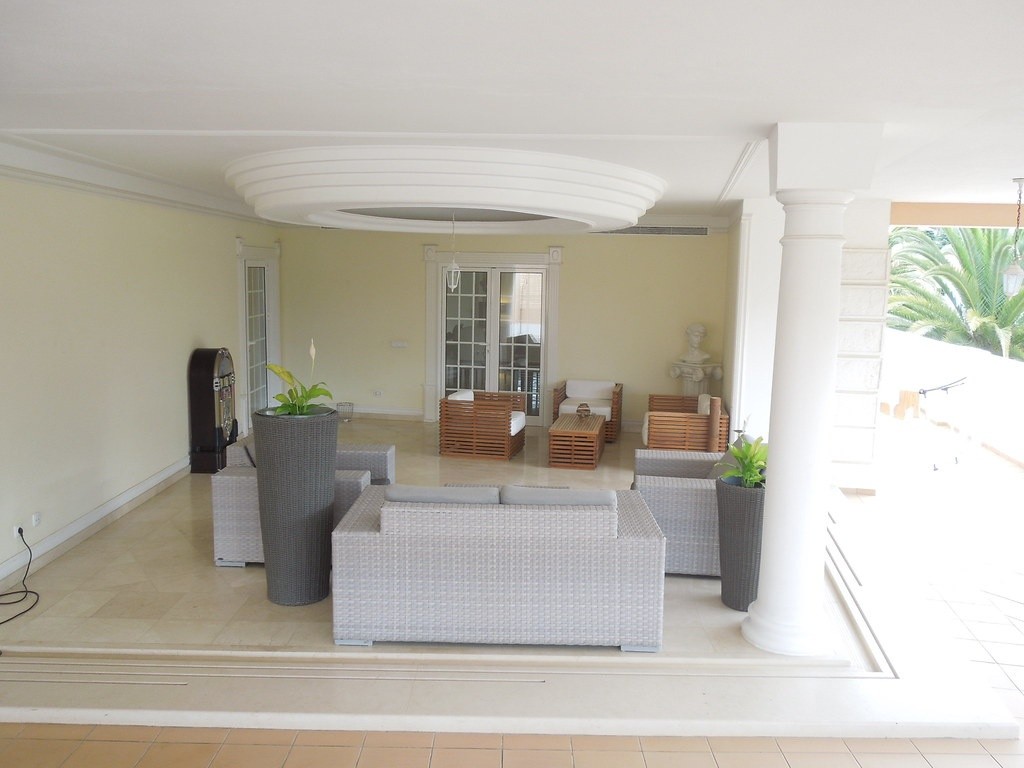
444 210 462 291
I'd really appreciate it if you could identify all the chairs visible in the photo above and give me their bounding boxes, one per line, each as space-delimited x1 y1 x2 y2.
641 394 730 452
438 390 527 462
552 379 623 441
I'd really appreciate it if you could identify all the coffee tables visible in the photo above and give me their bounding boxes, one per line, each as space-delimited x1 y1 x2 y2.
547 413 607 471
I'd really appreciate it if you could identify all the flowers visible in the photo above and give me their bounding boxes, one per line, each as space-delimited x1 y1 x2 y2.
251 337 333 415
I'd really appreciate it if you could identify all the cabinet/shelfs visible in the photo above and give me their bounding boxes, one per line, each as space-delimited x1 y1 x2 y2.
190 347 239 474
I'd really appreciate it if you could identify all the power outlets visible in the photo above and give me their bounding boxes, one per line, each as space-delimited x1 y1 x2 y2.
14 522 24 538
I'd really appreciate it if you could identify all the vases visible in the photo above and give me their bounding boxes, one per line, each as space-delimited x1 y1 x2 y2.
253 405 339 607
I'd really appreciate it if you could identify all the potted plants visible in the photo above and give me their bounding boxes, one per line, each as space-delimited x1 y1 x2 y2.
714 435 769 611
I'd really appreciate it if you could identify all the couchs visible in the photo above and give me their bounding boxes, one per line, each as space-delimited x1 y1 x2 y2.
332 483 666 651
212 433 395 568
632 436 756 576
